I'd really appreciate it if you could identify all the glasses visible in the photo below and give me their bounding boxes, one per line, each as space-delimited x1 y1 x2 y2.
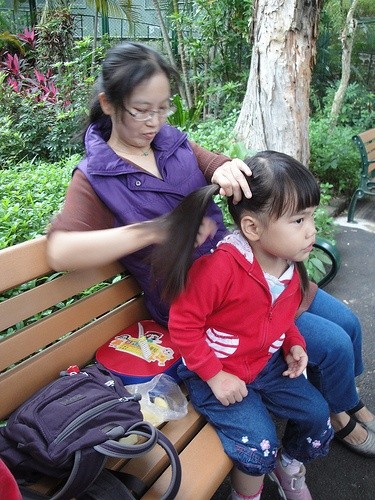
117 98 177 122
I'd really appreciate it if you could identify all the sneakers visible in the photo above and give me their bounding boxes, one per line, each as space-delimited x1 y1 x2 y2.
269 447 313 500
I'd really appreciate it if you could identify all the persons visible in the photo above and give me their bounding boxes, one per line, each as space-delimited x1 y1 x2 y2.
128 150 335 500
44 41 375 460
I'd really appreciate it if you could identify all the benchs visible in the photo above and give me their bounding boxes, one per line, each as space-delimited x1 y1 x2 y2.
348 128 375 221
1 235 342 500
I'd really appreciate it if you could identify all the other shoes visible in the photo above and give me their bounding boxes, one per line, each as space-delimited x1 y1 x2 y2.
328 414 375 456
349 400 375 430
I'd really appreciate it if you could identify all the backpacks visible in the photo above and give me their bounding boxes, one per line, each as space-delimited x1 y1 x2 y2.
1 362 183 499
96 317 190 399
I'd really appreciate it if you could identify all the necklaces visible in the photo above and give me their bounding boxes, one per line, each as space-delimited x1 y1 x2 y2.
108 138 152 156
